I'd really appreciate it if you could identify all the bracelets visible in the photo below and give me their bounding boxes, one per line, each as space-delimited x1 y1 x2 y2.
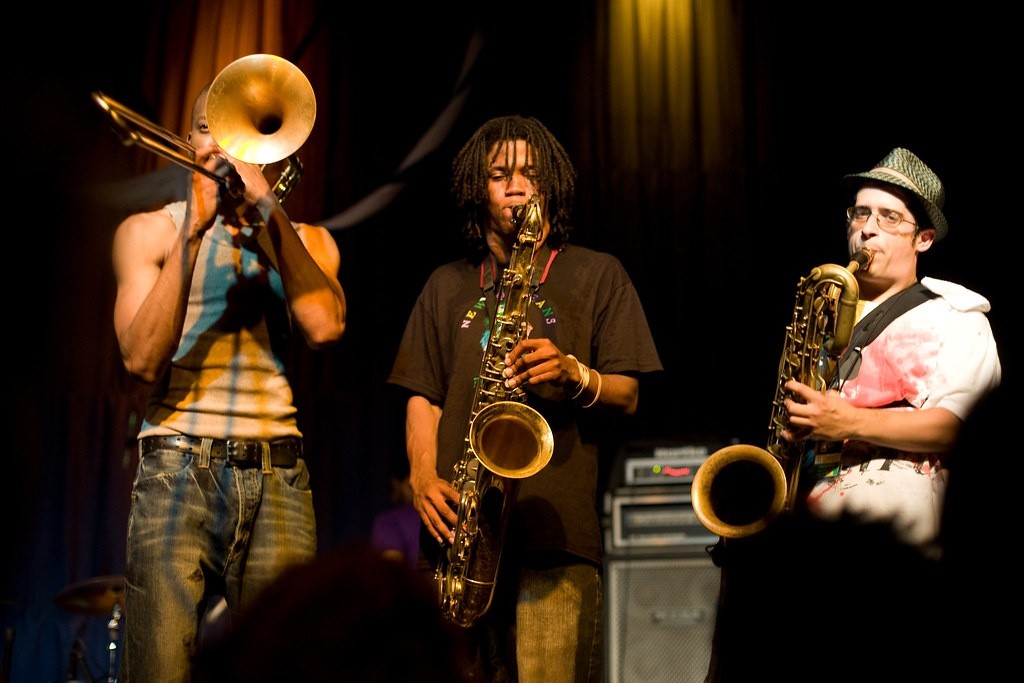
564 354 602 410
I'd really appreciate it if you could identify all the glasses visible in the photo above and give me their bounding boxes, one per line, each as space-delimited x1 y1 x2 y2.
846 205 921 232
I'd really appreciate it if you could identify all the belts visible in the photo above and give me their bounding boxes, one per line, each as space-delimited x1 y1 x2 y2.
140 432 304 467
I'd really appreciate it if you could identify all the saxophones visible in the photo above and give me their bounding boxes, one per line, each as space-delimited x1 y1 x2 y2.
431 195 555 628
691 246 873 539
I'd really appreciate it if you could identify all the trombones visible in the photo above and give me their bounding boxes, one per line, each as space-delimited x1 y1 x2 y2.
88 51 317 210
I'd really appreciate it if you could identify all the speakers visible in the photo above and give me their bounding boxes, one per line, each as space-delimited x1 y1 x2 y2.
601 557 724 682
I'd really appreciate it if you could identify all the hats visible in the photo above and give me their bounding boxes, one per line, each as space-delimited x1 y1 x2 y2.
843 147 948 241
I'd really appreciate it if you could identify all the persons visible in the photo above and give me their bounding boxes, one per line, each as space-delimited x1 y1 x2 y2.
711 147 1024 683
113 76 493 683
385 114 664 683
